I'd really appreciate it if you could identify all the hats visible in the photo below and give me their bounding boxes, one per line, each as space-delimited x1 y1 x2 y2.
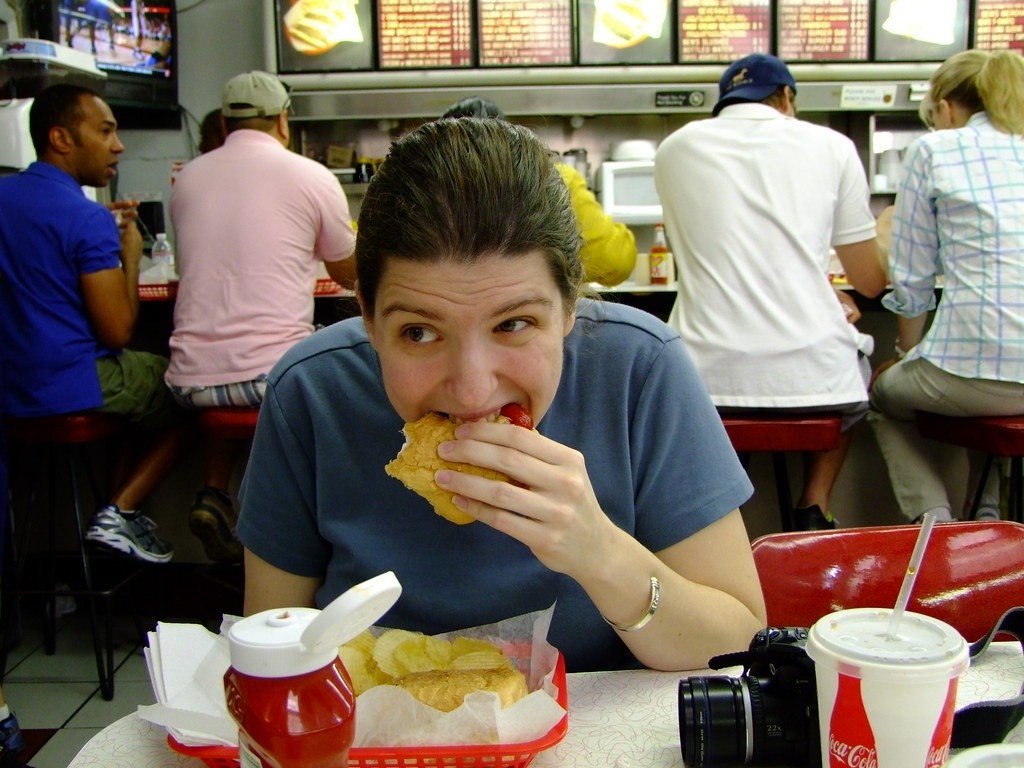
712 54 796 117
221 70 296 118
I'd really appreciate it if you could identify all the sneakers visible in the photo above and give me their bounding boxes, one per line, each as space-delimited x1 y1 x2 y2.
188 487 243 562
85 505 174 563
795 504 840 532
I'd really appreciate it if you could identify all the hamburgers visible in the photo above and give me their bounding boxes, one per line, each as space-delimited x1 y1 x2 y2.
386 404 537 525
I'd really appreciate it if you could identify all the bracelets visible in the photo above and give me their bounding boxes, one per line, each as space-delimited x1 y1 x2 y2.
600 577 660 632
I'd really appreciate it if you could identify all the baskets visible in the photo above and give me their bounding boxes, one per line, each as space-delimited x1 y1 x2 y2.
167 642 568 768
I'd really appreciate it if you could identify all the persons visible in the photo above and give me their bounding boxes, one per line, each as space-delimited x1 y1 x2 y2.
59 0 170 68
0 82 187 562
238 119 767 674
869 47 1023 524
654 52 895 532
164 71 355 564
444 96 638 298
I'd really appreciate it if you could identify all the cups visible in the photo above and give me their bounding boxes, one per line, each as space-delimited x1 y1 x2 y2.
804 607 971 768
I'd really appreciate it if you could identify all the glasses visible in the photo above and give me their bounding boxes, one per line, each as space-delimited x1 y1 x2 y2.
282 82 292 110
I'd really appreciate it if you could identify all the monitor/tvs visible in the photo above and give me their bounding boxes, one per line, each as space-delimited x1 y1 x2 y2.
26 0 180 104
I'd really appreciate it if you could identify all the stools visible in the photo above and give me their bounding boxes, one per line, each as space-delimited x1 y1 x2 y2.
958 416 1024 521
721 415 838 535
205 404 261 438
1 412 129 700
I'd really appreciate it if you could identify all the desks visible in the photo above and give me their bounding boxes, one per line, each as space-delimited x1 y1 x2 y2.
66 640 1024 768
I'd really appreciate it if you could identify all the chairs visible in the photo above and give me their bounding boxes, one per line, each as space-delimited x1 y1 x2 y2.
752 519 1024 644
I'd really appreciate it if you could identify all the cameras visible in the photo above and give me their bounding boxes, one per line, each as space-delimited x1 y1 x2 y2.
678 626 823 768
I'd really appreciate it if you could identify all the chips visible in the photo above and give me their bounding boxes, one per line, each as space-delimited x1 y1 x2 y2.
335 625 511 692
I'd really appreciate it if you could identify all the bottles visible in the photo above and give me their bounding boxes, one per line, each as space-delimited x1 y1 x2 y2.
152 232 175 279
223 569 404 768
649 224 667 286
